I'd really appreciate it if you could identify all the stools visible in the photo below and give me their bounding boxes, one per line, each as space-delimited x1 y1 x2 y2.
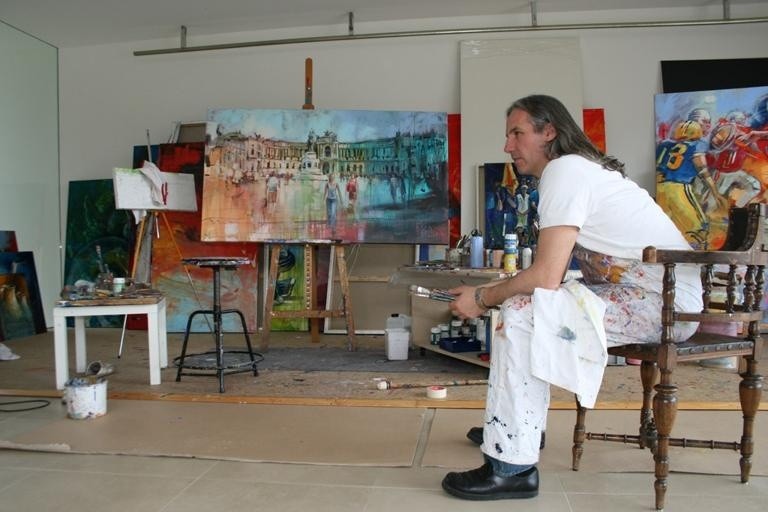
175 254 265 393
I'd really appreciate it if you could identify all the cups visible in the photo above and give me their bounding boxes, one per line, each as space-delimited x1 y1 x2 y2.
470 228 484 269
445 247 467 268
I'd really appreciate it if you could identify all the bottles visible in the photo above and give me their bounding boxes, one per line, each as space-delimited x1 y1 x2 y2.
112 277 126 297
431 317 487 346
522 244 532 270
504 233 517 274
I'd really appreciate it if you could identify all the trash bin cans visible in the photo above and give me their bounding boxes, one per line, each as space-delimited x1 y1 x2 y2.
386 328 409 361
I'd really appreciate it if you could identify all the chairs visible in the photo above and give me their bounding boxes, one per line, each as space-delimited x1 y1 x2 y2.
569 201 767 510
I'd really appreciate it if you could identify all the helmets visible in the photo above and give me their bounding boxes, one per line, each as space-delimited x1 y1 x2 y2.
668 108 745 144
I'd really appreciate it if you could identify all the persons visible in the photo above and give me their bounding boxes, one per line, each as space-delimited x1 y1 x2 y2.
340 171 407 212
441 96 705 500
223 171 294 213
656 109 768 250
486 181 539 249
323 173 343 236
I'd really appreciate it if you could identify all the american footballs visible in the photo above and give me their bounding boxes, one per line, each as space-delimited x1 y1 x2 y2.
712 123 737 148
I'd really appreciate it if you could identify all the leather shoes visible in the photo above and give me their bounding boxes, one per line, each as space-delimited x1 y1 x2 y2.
441 465 538 502
466 426 545 450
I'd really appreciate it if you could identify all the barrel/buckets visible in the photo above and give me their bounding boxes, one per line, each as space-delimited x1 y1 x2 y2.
387 313 411 331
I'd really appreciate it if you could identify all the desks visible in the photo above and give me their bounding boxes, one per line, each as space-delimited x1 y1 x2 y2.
400 264 582 378
51 296 169 390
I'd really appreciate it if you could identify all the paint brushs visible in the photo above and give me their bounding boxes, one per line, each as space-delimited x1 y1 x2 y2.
456 232 471 248
410 283 454 302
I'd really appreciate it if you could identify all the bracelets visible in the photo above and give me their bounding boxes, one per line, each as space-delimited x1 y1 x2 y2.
475 287 500 310
